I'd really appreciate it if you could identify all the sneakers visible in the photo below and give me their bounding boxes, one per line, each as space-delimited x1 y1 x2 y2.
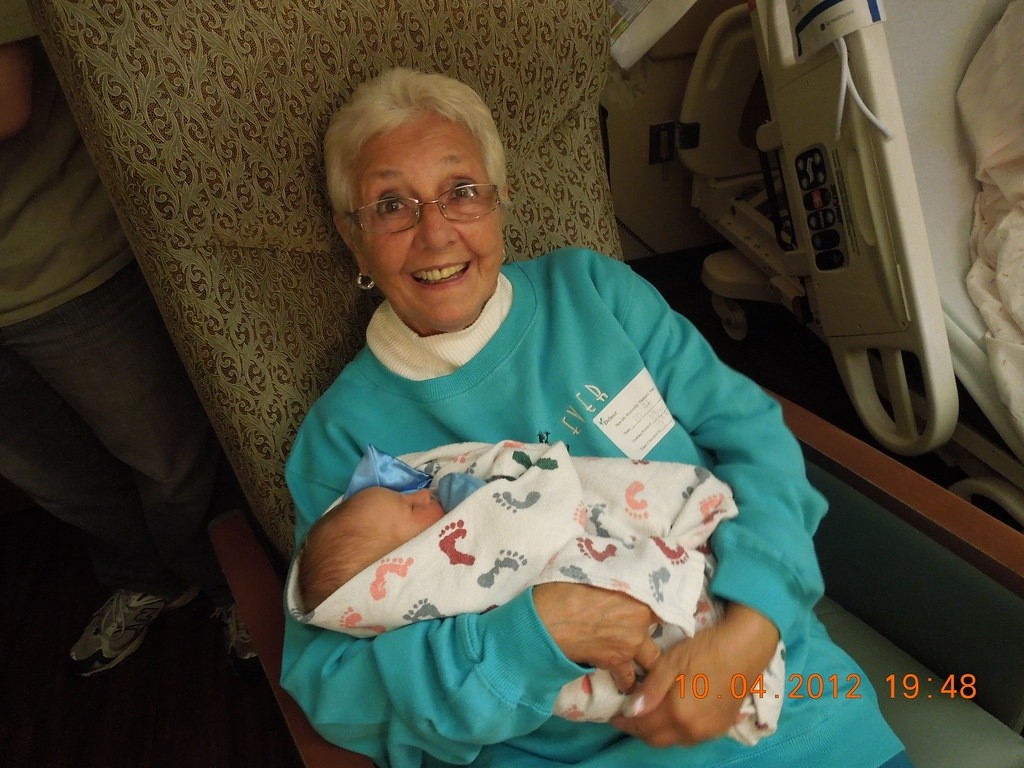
209 603 259 670
67 586 201 679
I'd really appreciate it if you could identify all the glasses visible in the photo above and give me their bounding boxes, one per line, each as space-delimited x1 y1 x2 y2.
344 182 502 235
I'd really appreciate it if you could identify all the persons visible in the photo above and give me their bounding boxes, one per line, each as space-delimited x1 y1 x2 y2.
295 430 793 737
278 64 916 768
1 0 262 680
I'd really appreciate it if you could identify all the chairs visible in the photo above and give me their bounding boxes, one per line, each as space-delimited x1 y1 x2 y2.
32 0 1024 768
